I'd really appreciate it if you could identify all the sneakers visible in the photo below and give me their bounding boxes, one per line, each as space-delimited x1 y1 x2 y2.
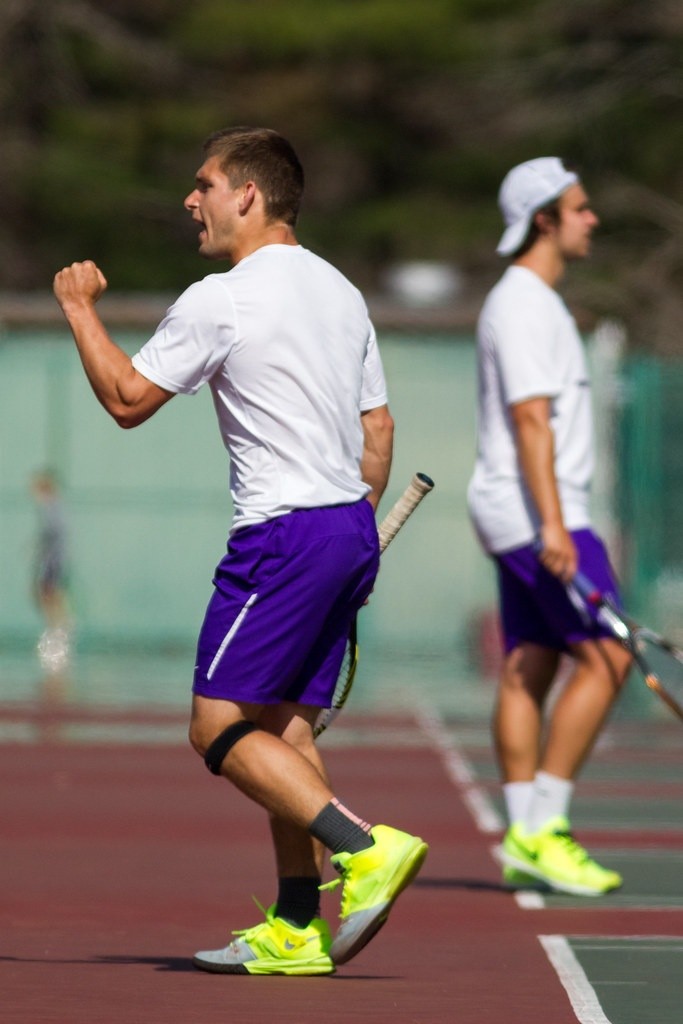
196 895 337 976
317 824 430 963
503 818 623 898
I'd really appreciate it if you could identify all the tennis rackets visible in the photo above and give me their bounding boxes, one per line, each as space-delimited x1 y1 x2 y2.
310 472 435 741
530 537 683 720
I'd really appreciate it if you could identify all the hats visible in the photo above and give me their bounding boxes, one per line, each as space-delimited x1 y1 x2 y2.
497 156 578 255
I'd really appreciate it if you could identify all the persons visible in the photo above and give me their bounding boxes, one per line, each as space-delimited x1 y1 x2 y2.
466 157 638 897
52 128 430 975
